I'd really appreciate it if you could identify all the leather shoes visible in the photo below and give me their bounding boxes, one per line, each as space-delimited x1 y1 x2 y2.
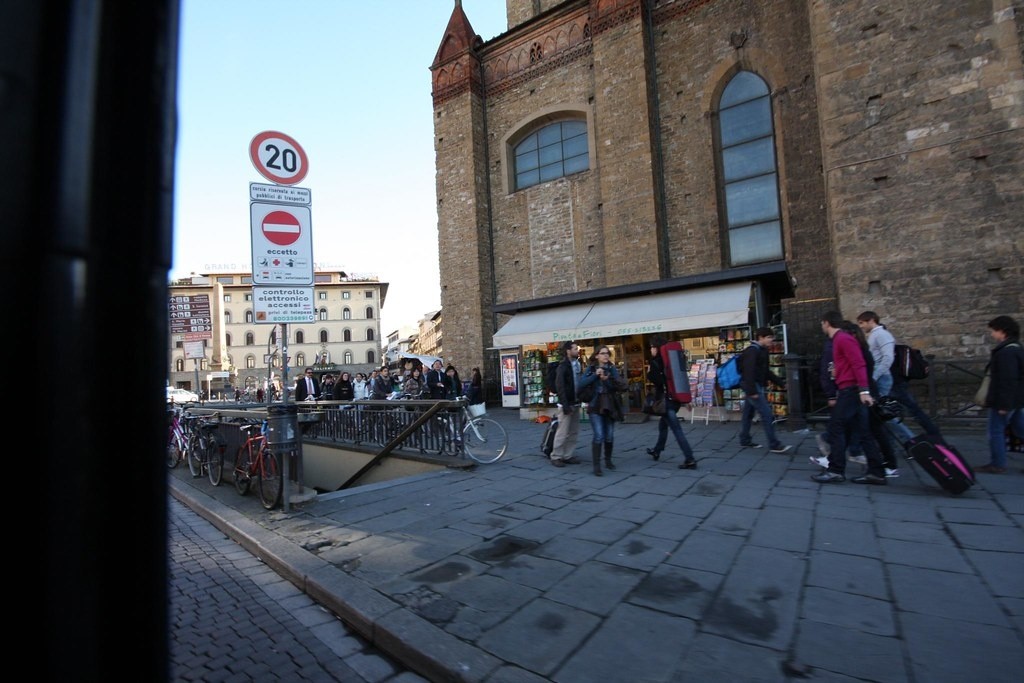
851 475 887 485
811 471 844 482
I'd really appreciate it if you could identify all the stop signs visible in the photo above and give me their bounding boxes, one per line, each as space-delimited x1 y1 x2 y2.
262 211 301 245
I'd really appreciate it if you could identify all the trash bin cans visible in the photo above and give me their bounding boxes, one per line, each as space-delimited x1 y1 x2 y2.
783 354 822 415
266 403 299 454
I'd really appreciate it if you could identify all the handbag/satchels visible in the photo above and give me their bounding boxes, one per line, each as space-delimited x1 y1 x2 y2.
653 398 666 413
973 375 993 407
577 366 596 402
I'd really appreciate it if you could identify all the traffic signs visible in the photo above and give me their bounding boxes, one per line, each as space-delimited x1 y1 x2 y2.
168 294 212 332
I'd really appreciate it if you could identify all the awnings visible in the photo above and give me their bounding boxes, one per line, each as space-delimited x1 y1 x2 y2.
493 277 759 348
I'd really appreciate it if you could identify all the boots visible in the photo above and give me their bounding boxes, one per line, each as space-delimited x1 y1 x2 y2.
604 442 615 469
592 442 604 475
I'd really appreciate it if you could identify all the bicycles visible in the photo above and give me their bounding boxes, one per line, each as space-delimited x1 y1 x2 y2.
414 396 510 464
233 421 283 510
166 396 226 486
306 394 445 455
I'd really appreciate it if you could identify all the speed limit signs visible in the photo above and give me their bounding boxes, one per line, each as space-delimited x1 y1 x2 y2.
248 130 309 186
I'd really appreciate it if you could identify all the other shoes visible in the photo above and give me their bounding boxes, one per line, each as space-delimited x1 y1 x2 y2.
647 448 660 460
770 445 791 453
979 463 1008 474
680 458 696 468
553 460 565 467
564 457 580 464
740 443 762 448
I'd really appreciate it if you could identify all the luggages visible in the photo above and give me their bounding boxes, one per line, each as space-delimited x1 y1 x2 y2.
541 417 558 456
870 405 975 494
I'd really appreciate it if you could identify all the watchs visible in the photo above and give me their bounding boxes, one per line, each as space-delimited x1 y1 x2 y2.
858 390 870 395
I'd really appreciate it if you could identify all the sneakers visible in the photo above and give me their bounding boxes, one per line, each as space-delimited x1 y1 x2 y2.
810 455 830 468
885 468 899 478
848 456 868 464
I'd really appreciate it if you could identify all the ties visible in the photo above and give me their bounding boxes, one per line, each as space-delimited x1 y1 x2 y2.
308 379 312 394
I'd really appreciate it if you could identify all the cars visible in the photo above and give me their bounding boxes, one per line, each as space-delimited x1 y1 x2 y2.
167 389 198 402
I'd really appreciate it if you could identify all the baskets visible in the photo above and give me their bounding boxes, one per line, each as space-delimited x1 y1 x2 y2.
469 402 485 417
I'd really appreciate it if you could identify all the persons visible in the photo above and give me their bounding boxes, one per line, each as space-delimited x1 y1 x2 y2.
854 311 896 403
578 346 628 477
809 311 950 477
550 341 582 467
737 327 793 453
295 360 485 426
469 367 482 405
644 335 698 469
974 316 1023 473
627 369 645 409
811 311 888 485
231 383 278 402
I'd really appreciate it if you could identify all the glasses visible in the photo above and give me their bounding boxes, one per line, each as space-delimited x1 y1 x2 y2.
306 372 313 374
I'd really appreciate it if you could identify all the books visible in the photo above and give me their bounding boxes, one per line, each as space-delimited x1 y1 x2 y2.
684 363 718 407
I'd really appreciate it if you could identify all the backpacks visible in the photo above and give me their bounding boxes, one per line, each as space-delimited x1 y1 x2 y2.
545 362 560 404
716 345 760 389
898 345 930 379
